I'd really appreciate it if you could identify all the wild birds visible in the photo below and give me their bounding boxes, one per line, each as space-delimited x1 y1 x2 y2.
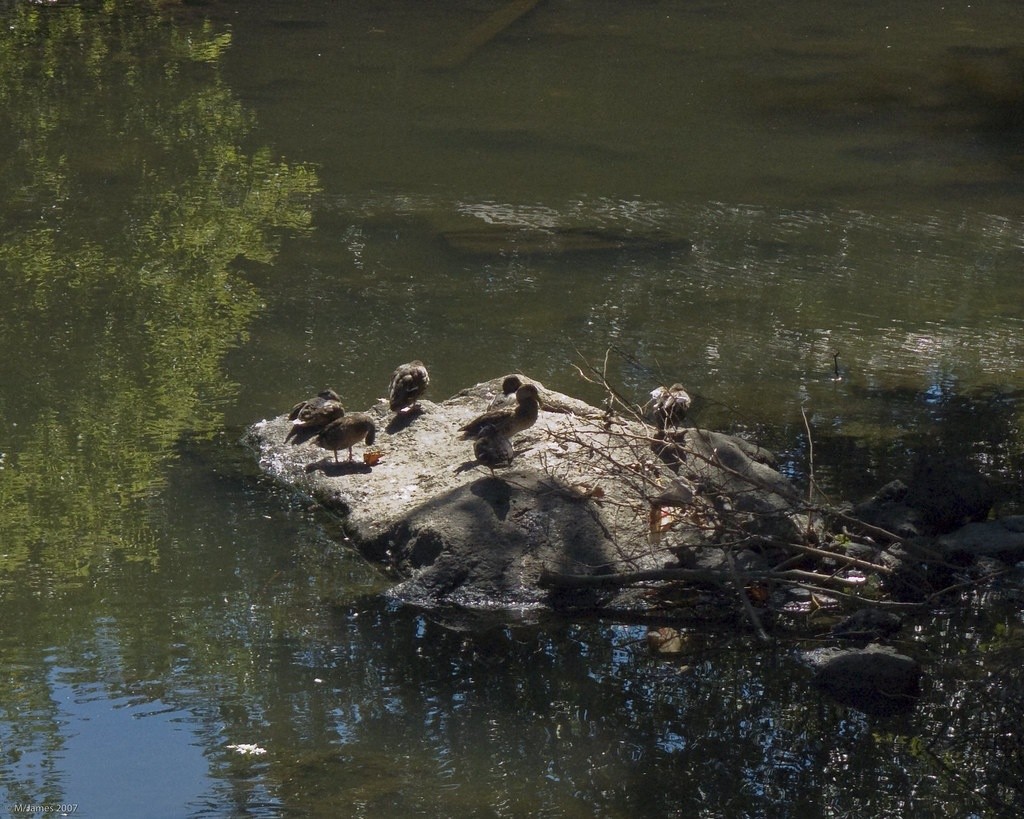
281 358 709 549
638 625 691 654
